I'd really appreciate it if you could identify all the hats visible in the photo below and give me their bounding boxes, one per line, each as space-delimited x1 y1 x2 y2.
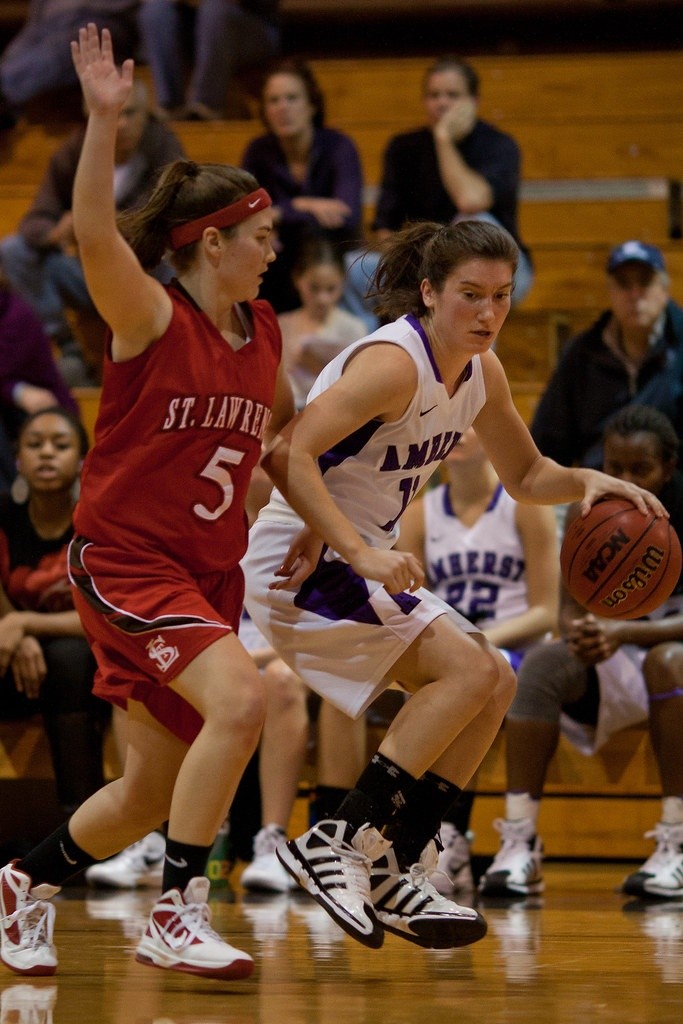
606 241 663 270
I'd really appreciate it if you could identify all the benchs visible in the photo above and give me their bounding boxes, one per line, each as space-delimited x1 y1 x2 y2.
1 50 683 868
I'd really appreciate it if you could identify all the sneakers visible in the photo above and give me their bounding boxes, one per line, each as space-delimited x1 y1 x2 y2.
276 820 393 949
481 818 545 894
241 826 291 894
369 839 488 950
621 820 683 895
136 877 255 979
1 859 57 976
428 822 473 893
86 831 166 889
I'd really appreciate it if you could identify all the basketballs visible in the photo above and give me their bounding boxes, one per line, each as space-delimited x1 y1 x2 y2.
558 498 683 622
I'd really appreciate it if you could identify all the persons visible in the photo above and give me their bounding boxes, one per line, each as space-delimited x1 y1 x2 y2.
84 610 315 890
483 404 682 899
0 0 286 457
1 409 118 810
342 57 533 333
239 55 361 316
529 241 683 471
240 221 672 950
277 237 368 413
1 22 295 982
313 427 561 895
